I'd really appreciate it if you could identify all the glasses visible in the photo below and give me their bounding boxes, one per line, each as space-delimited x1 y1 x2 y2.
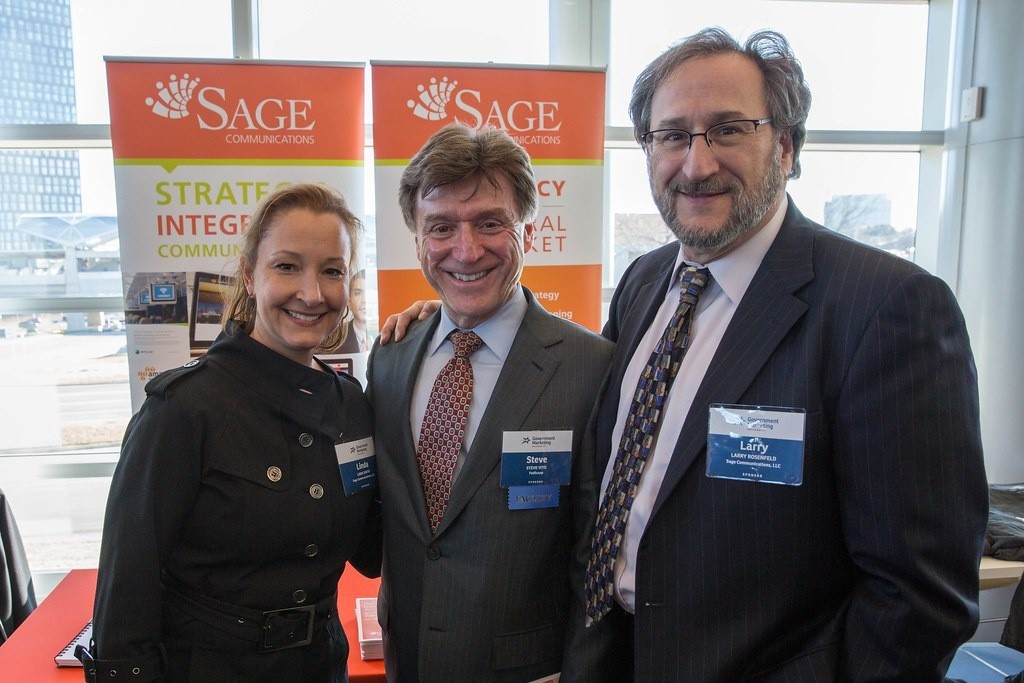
641 117 773 161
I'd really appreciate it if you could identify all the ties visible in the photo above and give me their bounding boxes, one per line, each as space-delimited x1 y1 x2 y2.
415 329 486 536
581 262 713 629
361 337 367 352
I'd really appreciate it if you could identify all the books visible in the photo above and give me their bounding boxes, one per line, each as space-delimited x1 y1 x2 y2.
355 598 386 660
54 618 95 669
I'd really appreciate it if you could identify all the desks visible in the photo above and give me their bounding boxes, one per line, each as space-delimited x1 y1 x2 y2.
978 556 1024 590
0 559 386 683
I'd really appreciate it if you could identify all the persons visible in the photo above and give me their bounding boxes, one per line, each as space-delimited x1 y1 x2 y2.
335 270 367 354
74 183 382 683
363 122 617 683
379 26 989 683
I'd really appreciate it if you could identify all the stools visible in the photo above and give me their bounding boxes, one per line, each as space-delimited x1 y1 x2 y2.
944 643 1024 683
